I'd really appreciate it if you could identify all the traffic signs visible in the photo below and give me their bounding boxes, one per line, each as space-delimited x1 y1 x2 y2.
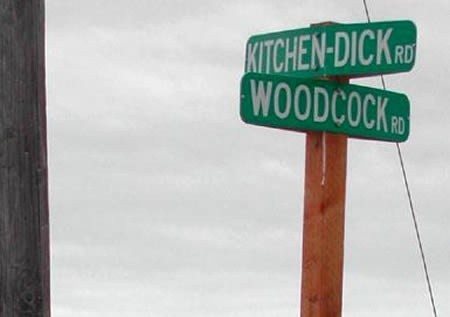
245 21 416 76
240 72 409 143
